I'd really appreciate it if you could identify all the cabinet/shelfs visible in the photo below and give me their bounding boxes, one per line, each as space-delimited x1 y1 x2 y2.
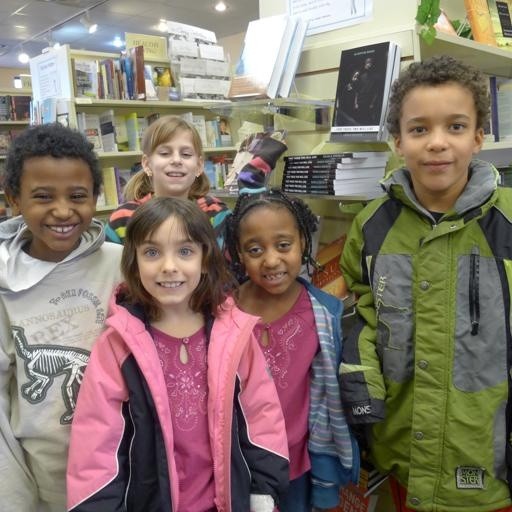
0 31 512 229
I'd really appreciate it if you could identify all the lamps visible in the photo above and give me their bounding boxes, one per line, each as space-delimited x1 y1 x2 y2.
79 17 98 35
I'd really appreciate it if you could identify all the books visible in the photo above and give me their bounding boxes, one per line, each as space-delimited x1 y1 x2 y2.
482 134 495 143
464 1 511 53
1 31 402 203
495 165 511 187
336 444 391 512
310 232 358 326
487 74 511 142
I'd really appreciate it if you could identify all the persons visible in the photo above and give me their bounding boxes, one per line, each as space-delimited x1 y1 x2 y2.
222 184 366 511
335 53 512 512
0 122 127 512
102 114 289 297
66 194 292 511
